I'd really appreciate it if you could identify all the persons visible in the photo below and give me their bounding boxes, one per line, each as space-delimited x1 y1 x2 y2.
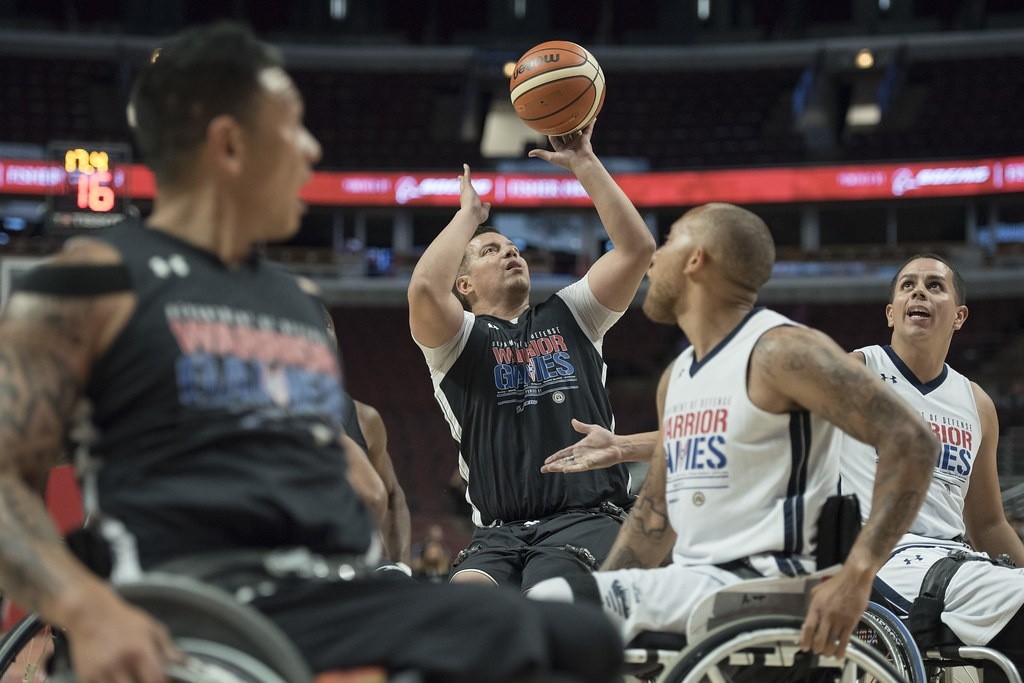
0 24 656 683
527 202 1024 683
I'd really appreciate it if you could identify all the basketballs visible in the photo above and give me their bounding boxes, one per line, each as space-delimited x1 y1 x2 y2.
510 41 606 136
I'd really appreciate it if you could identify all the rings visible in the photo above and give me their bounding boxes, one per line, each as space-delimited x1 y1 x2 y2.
829 633 840 646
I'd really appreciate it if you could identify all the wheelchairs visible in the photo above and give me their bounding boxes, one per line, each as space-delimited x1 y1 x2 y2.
0 441 1024 683
